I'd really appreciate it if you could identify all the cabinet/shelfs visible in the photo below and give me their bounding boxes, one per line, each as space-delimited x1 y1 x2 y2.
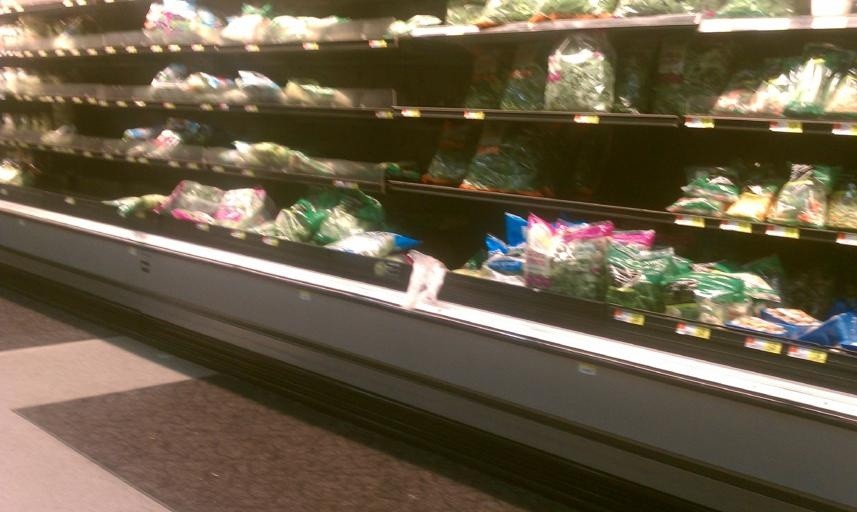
0 2 857 510
